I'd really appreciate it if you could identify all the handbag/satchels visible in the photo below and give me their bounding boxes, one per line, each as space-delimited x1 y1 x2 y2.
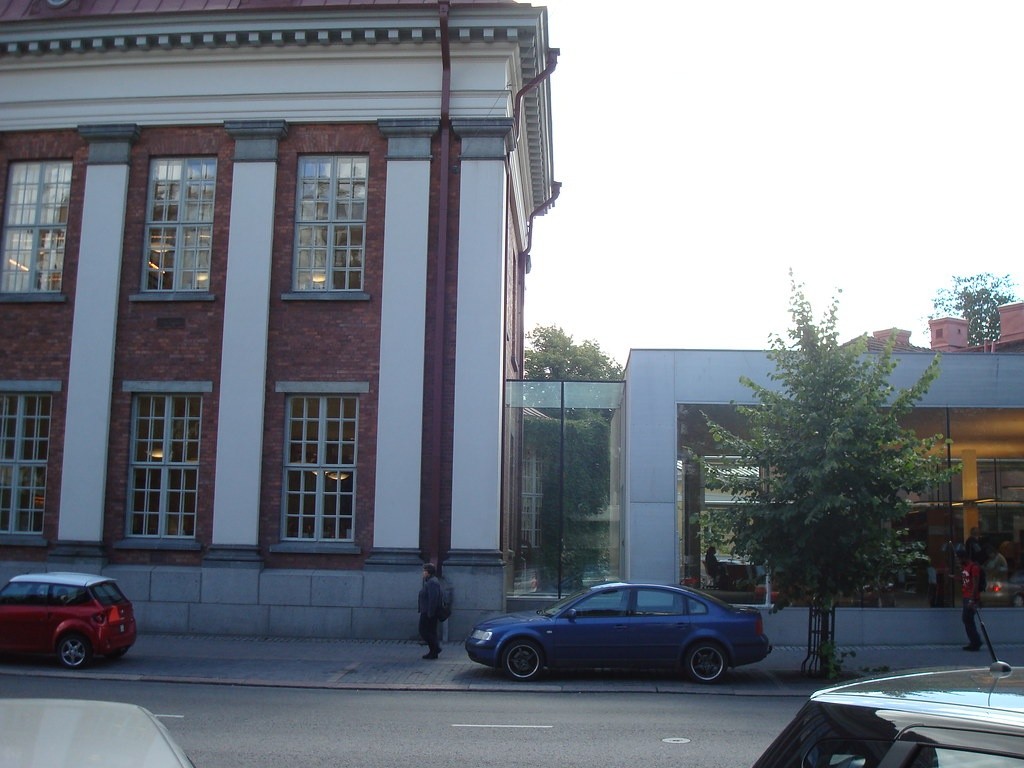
439 607 451 622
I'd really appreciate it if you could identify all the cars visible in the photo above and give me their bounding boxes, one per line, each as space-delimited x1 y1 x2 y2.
466 583 772 683
750 666 1024 768
934 540 1023 607
0 572 137 670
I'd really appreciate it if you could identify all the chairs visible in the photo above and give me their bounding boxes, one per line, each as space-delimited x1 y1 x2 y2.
56 589 69 602
702 561 719 589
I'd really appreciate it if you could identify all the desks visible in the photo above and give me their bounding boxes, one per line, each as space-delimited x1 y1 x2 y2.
720 562 758 591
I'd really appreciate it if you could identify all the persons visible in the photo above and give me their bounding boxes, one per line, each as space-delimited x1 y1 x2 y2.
704 545 725 577
949 548 983 651
416 563 442 659
966 527 989 564
980 536 1015 594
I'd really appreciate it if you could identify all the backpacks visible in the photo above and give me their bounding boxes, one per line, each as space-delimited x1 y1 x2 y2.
971 564 986 592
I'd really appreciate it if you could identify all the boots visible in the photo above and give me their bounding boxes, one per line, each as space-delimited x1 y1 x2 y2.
422 643 442 659
962 621 982 651
